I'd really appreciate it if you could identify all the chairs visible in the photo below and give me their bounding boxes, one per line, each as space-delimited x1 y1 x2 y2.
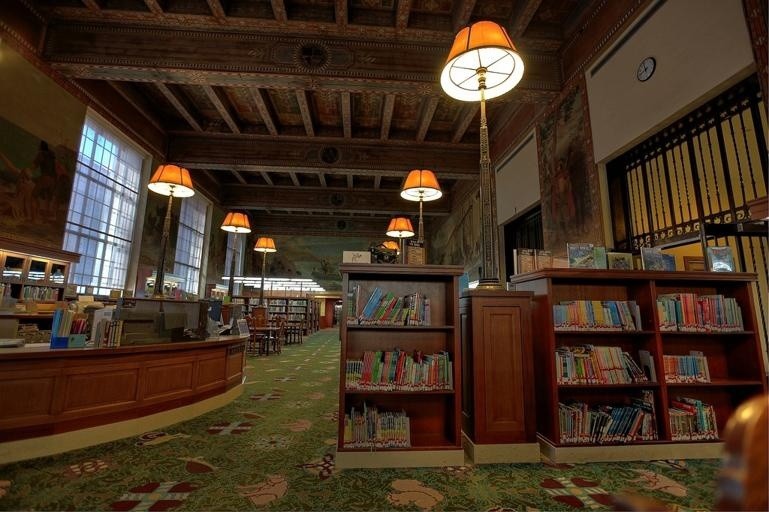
244 312 305 357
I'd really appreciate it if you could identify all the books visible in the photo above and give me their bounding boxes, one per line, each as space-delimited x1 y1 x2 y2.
344 284 453 449
466 278 507 291
0 282 133 351
220 295 318 336
133 281 201 301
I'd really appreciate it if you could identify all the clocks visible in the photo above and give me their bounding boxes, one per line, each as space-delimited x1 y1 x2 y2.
635 57 657 83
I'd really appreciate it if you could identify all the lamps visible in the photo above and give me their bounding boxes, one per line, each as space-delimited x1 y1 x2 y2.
398 166 445 265
379 242 398 255
253 237 278 304
385 217 417 264
145 162 198 300
216 210 252 299
440 19 525 291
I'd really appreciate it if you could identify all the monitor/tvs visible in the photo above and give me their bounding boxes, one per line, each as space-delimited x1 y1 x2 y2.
198 300 222 321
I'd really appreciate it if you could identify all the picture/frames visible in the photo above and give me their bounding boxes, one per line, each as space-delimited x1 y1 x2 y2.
511 239 739 270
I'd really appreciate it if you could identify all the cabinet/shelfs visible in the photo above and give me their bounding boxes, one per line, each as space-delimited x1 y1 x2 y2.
1 230 83 318
333 259 467 473
508 264 768 463
231 295 320 336
